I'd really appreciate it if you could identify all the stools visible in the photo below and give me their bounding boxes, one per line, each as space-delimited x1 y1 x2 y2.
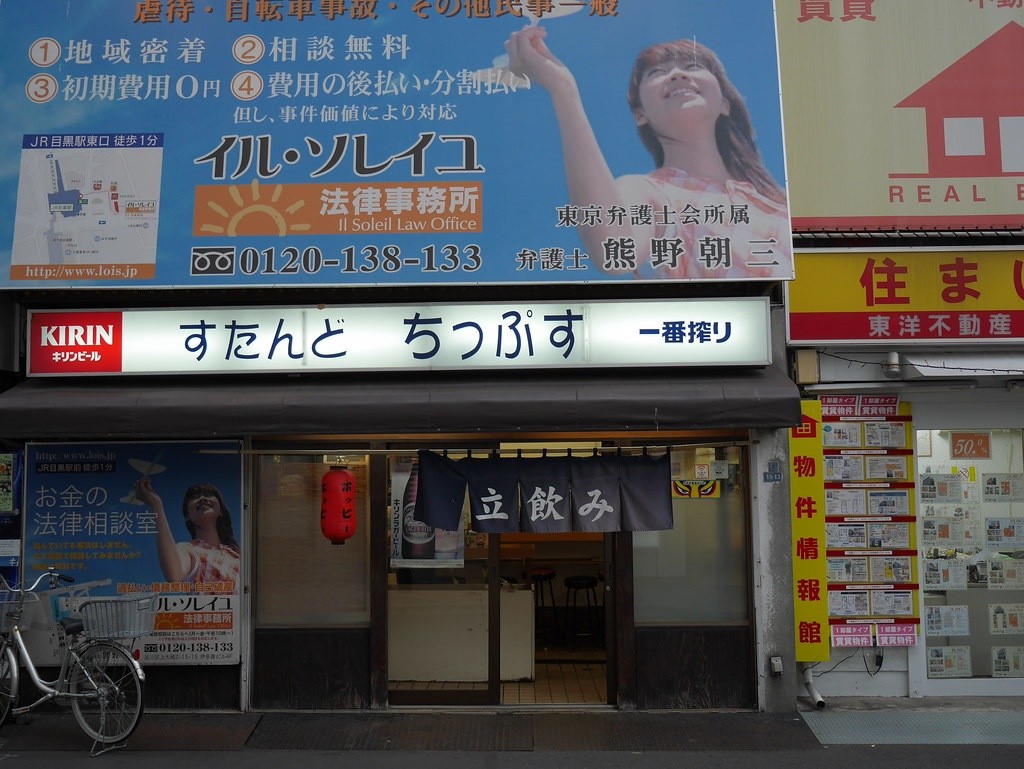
521 566 563 648
564 575 604 653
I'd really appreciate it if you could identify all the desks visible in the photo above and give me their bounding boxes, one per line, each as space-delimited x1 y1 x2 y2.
464 547 604 561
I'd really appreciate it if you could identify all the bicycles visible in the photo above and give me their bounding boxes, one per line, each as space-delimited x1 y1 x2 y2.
0 566 158 759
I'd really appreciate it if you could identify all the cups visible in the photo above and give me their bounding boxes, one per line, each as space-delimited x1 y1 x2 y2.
388 573 397 585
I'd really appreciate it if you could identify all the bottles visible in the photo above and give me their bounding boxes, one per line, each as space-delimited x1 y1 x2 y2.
401 458 435 559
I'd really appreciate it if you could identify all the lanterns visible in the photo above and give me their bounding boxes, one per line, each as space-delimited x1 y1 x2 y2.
321 466 356 545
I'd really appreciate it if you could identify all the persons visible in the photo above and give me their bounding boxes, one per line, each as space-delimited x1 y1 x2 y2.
134 479 241 594
504 26 792 279
